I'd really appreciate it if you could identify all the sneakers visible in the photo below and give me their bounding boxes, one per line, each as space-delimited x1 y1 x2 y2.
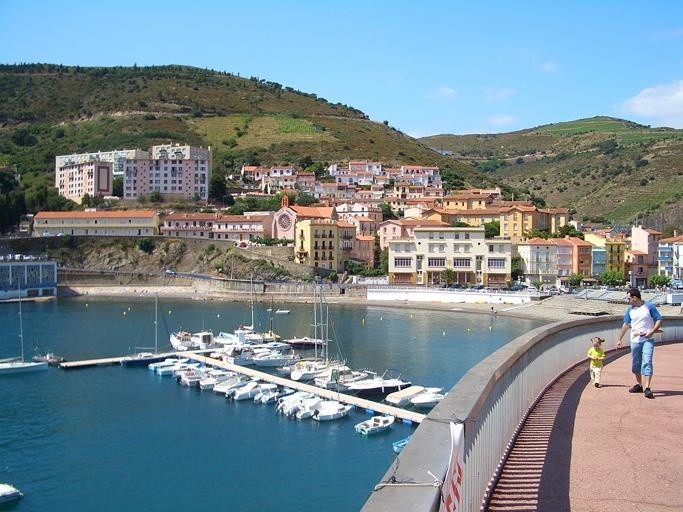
644 387 653 398
595 383 600 388
628 383 643 394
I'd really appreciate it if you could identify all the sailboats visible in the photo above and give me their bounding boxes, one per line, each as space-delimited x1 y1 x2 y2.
0 351 62 374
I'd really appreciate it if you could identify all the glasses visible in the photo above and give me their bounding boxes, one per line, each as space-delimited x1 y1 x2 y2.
627 295 635 300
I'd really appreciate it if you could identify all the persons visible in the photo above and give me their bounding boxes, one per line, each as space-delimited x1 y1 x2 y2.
587 336 606 388
616 288 662 397
